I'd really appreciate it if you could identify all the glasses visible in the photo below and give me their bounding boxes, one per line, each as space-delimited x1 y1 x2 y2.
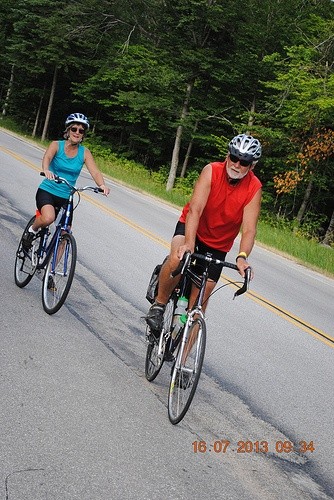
230 151 250 166
70 126 85 134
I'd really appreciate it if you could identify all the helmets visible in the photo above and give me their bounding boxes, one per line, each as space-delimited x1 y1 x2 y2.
66 113 89 132
229 133 261 162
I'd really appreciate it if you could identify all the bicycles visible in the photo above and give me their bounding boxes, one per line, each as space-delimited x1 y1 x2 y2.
15 171 107 315
143 249 252 425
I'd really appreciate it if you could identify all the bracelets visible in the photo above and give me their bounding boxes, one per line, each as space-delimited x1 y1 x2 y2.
239 252 248 258
99 184 102 187
236 256 246 262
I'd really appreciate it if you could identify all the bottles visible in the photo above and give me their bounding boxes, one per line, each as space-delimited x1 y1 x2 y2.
46 221 56 248
172 296 188 327
171 315 187 340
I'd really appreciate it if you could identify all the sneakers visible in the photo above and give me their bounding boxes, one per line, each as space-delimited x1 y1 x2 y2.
43 275 55 288
22 224 35 248
146 302 165 329
171 366 189 387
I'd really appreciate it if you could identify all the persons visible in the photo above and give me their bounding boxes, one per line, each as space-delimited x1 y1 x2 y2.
22 113 110 291
146 134 261 391
